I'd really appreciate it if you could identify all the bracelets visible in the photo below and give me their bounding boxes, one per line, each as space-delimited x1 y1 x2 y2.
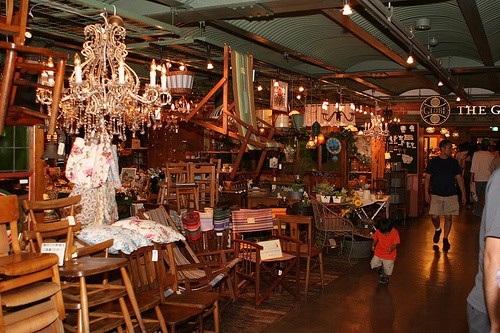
462 193 465 194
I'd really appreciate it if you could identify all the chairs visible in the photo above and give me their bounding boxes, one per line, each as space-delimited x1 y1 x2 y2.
0 158 355 333
0 0 70 142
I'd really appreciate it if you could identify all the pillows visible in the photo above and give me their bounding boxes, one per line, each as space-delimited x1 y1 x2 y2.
112 218 186 244
75 225 154 254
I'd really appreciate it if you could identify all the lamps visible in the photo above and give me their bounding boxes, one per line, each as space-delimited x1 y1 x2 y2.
321 88 390 141
343 0 352 16
37 12 172 154
407 50 413 64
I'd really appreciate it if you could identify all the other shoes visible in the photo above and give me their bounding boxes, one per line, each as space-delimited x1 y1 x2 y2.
377 266 383 274
443 238 450 249
379 273 391 284
433 228 442 243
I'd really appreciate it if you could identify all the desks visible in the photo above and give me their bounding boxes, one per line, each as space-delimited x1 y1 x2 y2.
354 194 392 231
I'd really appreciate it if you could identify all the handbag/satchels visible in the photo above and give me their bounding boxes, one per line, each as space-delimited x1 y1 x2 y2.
469 182 478 203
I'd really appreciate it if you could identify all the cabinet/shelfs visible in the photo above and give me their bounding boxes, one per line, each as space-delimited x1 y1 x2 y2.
348 171 407 227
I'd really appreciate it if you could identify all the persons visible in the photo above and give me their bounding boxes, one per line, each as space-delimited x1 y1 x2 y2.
469 141 500 216
466 167 500 333
370 218 400 283
425 140 466 246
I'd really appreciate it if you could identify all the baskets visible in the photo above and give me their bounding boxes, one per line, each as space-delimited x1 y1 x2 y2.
167 57 194 93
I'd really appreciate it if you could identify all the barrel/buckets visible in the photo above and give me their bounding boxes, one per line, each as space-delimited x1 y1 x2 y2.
344 240 370 258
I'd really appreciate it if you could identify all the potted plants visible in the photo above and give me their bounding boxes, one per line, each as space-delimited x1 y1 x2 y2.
290 183 302 199
331 191 345 204
312 180 335 203
